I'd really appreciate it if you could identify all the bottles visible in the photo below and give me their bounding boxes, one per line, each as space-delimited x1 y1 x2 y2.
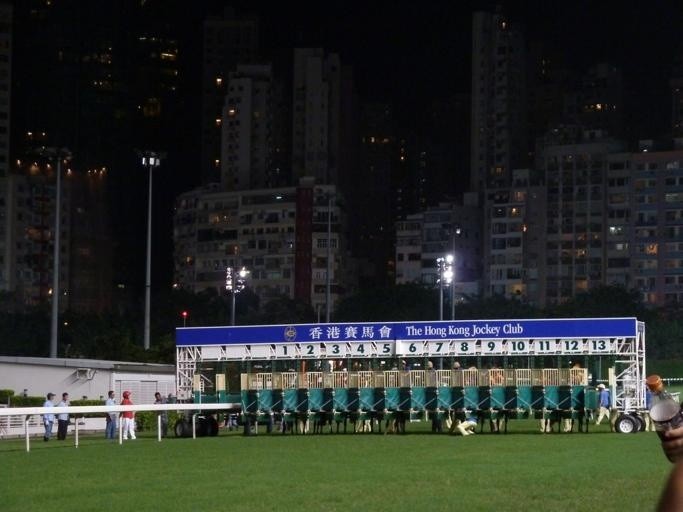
643 374 683 464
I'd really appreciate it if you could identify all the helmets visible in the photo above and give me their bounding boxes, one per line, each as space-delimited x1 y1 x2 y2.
123 391 131 399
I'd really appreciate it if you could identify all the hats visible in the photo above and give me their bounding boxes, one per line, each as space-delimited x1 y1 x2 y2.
48 393 57 400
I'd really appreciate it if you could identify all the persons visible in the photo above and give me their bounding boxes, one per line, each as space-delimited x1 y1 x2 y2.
595 383 612 425
644 385 656 432
657 411 683 512
42 393 55 441
118 391 137 440
56 393 68 440
104 391 118 440
153 392 169 436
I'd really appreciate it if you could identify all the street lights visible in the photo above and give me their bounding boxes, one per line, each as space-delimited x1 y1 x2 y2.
436 252 454 321
42 143 64 359
449 221 465 320
224 264 250 327
134 148 163 352
